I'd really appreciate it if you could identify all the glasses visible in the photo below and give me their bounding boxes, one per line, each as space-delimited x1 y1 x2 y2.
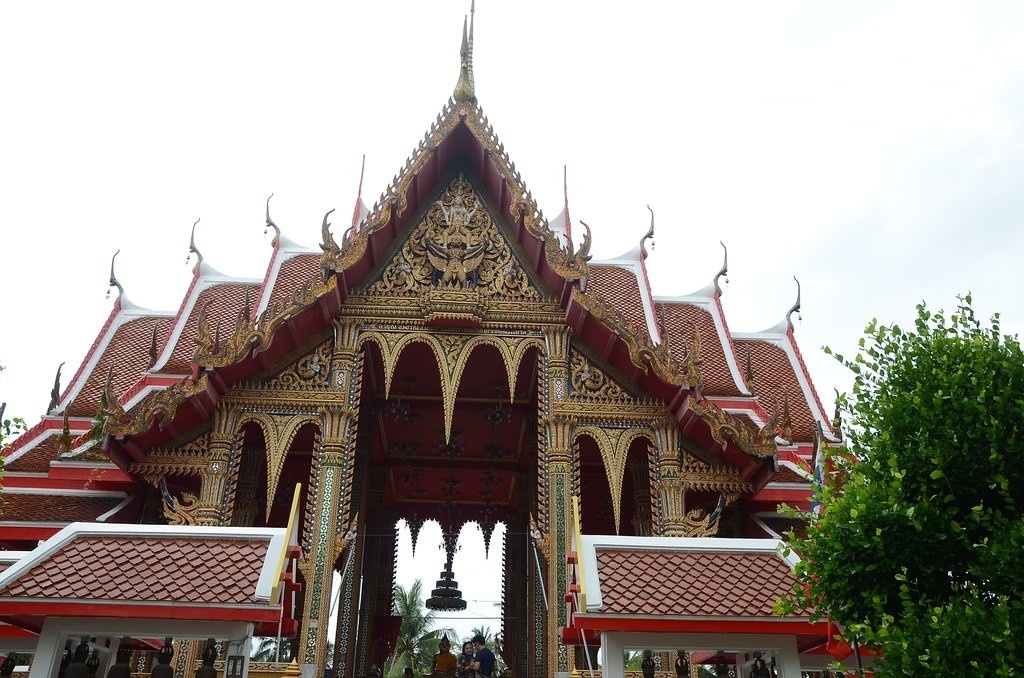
474 642 477 646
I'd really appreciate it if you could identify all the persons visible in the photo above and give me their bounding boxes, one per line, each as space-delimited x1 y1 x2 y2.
458 642 478 678
715 649 730 678
426 236 487 291
445 170 477 206
195 638 218 678
151 637 175 678
642 650 655 678
60 635 90 678
0 651 18 678
750 652 772 678
107 636 132 678
437 197 482 251
674 650 690 677
472 635 498 678
432 633 459 678
